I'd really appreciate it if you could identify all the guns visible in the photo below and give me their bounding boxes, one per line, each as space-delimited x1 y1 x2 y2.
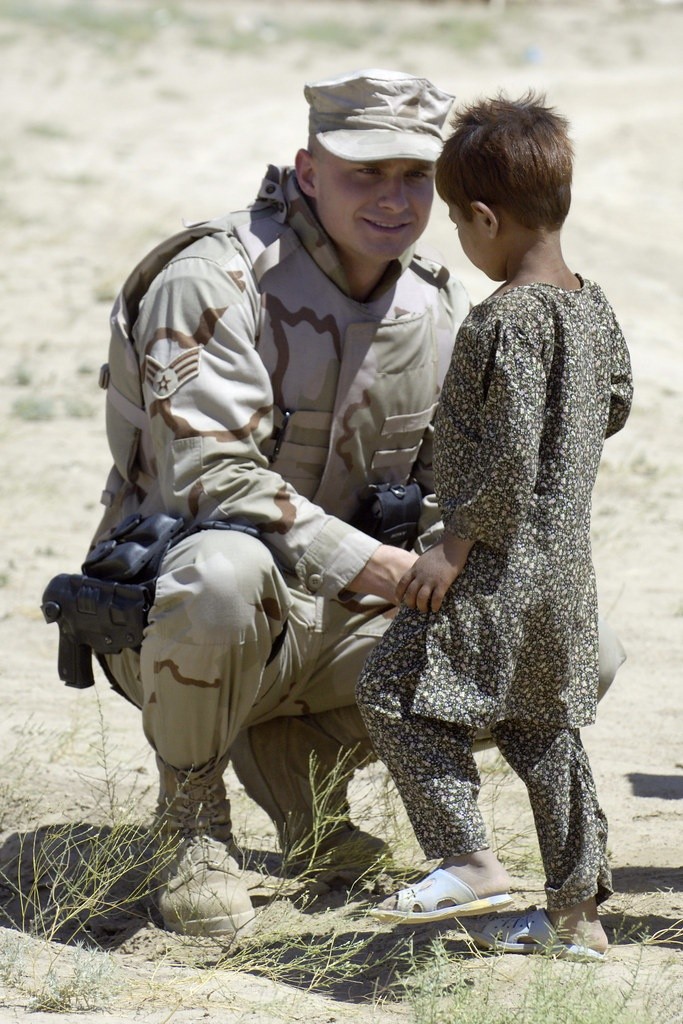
39 572 152 690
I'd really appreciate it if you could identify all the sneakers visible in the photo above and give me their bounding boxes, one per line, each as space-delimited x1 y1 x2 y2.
227 715 395 882
153 762 256 942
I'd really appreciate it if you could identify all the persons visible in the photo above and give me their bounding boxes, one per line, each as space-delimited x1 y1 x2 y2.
77 73 625 945
355 96 633 962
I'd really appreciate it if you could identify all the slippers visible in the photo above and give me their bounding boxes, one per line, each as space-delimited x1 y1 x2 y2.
468 911 621 968
364 867 526 924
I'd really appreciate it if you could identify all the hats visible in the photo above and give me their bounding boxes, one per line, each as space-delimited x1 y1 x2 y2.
303 66 458 163
